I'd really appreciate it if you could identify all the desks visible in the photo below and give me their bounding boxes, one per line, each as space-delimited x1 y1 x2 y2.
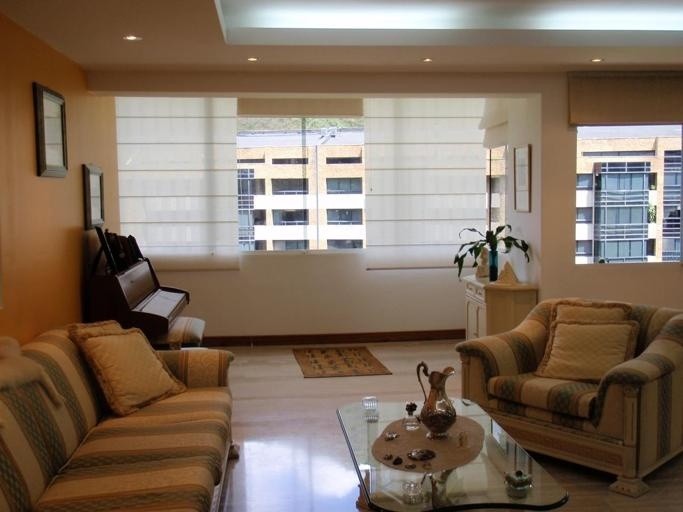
336 397 570 512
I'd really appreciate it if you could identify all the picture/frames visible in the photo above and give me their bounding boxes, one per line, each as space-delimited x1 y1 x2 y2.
32 83 69 178
82 164 106 231
514 144 531 213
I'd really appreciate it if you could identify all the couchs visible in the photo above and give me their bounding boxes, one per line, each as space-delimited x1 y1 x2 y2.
455 297 683 498
0 325 240 512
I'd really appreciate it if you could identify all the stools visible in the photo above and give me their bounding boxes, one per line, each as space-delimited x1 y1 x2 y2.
154 316 205 349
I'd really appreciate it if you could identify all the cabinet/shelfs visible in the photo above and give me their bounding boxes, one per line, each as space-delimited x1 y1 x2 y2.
462 275 539 341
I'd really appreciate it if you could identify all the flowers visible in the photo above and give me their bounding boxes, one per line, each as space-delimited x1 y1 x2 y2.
648 207 656 222
454 224 530 282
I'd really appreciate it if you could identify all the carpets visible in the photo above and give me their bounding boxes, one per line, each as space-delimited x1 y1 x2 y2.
292 346 392 378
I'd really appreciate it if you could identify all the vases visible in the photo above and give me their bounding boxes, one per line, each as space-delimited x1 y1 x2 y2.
489 251 498 280
650 184 655 189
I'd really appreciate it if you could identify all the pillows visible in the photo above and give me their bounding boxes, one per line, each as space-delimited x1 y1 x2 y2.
69 320 122 344
82 328 187 416
551 300 631 323
534 321 640 384
